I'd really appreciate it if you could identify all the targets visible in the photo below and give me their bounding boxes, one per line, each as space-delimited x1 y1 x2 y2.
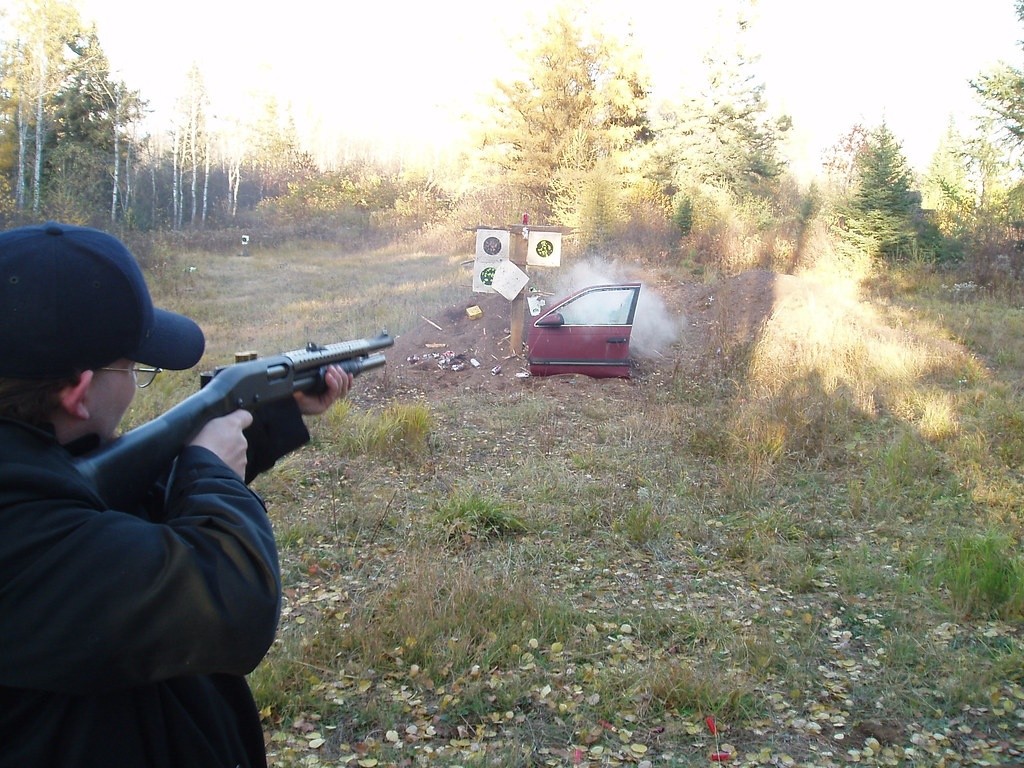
471 261 528 302
475 228 510 261
527 231 561 267
241 235 249 244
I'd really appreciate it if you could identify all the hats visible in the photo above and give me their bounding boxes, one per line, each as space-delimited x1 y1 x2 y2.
0 221 206 376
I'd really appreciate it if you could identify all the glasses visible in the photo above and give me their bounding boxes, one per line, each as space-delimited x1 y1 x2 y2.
100 363 164 389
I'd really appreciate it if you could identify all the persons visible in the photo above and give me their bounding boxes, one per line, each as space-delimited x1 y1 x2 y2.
0 223 353 768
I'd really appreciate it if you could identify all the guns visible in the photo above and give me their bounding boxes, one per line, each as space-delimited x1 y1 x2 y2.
64 329 398 493
